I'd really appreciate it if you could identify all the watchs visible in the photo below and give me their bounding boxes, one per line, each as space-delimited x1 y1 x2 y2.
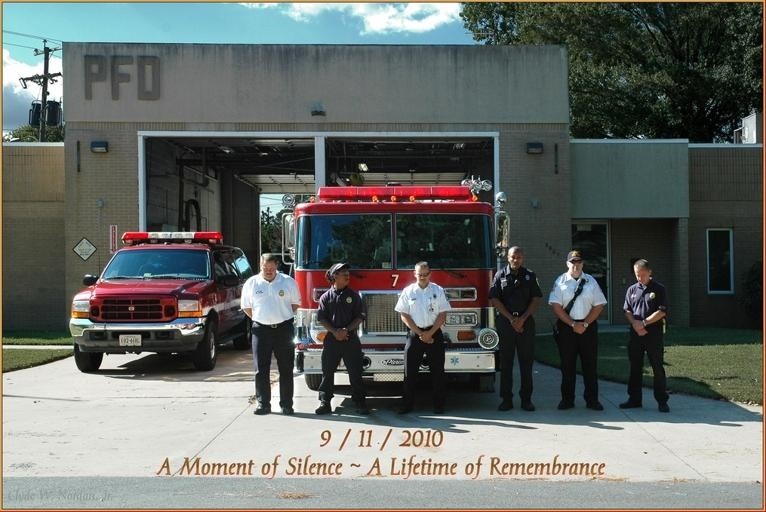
582 321 589 328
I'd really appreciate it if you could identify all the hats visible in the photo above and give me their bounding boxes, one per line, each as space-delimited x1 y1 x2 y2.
332 263 351 274
567 250 583 261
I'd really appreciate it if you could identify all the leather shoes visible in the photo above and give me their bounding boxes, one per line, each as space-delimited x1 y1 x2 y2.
255 404 271 414
281 405 293 415
659 400 669 411
587 401 603 410
558 400 574 408
316 404 331 414
398 407 412 413
499 400 513 409
358 405 369 414
521 400 535 411
434 406 443 413
619 399 641 408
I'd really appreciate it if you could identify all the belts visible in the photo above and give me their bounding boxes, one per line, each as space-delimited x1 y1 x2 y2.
255 318 293 328
510 312 523 316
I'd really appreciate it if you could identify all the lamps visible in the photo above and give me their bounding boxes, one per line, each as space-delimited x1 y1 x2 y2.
525 142 544 155
90 140 109 153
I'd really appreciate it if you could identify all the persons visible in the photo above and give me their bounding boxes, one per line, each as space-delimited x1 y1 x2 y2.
547 249 608 411
241 253 301 415
315 262 369 413
619 258 670 413
488 245 543 412
394 261 452 415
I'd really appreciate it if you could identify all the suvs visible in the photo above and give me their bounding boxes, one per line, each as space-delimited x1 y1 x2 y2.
63 226 256 378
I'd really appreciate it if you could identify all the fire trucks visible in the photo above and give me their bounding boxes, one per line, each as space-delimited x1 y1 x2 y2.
273 172 514 397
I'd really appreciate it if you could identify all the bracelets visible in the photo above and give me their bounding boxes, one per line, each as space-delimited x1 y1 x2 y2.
510 318 515 324
642 319 646 327
343 327 349 331
572 321 576 328
